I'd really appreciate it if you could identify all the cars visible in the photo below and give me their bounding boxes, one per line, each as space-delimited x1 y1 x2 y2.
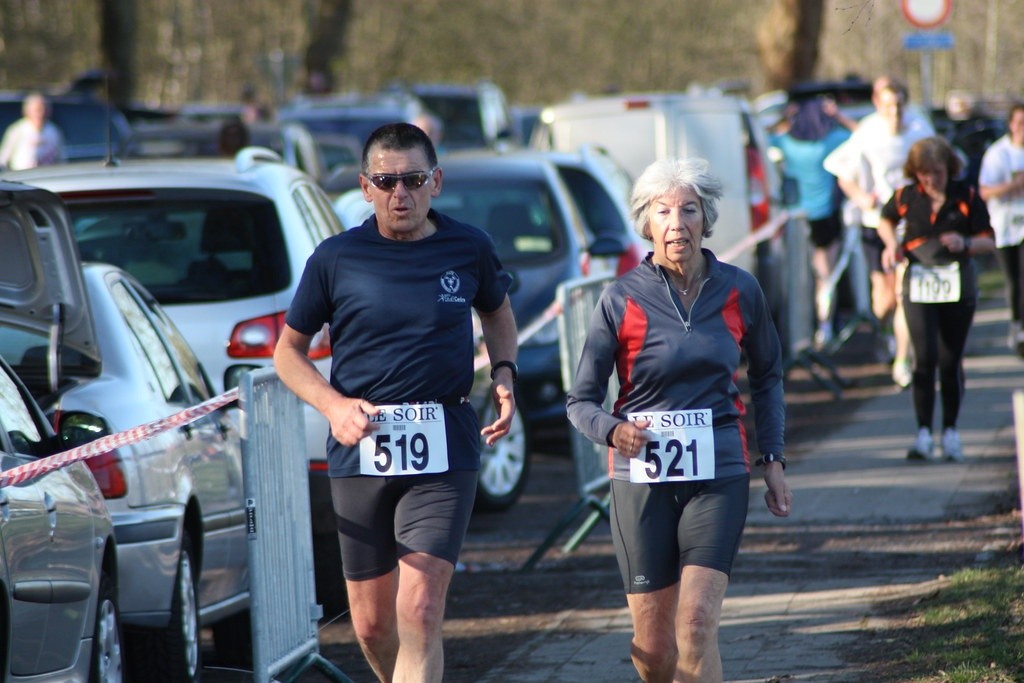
0 79 871 683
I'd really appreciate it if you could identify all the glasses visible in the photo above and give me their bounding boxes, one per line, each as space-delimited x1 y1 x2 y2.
365 166 437 191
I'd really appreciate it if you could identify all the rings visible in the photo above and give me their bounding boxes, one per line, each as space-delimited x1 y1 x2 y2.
629 438 635 452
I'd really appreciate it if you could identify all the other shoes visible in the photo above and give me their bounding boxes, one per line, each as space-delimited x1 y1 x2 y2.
907 426 933 461
1008 320 1024 349
941 427 963 463
892 355 913 388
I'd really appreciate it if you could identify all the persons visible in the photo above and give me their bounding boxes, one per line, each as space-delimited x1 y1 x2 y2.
411 114 443 148
565 158 794 683
943 89 997 184
977 105 1024 349
878 136 996 461
238 83 271 122
271 123 517 683
767 96 860 342
822 76 936 389
0 91 63 171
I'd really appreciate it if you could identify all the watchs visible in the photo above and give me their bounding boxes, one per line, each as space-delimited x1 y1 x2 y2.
490 360 519 383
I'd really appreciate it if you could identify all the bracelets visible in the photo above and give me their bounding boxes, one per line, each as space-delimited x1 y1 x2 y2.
755 454 787 470
964 238 971 250
